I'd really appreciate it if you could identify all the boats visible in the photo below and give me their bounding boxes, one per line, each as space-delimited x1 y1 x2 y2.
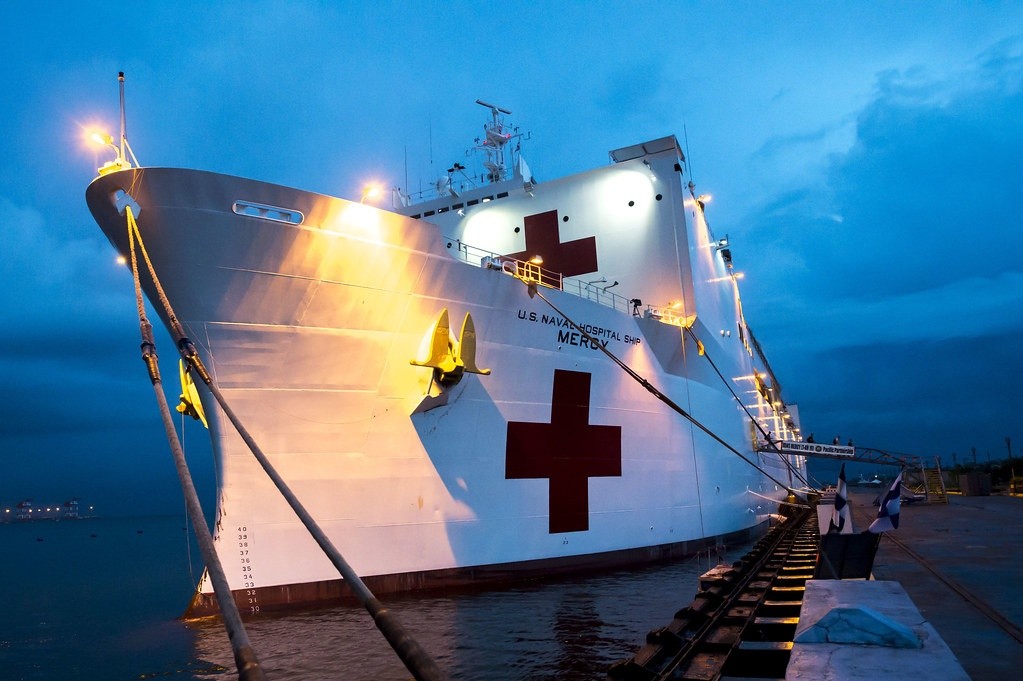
15 497 98 523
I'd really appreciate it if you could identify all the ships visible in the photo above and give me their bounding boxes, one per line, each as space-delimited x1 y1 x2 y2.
85 99 809 625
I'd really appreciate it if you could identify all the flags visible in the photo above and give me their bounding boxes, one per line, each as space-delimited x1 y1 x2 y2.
829 463 853 534
868 471 902 533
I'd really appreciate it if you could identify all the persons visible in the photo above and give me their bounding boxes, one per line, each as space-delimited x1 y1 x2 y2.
766 431 771 439
806 433 815 442
848 439 853 446
832 436 840 444
